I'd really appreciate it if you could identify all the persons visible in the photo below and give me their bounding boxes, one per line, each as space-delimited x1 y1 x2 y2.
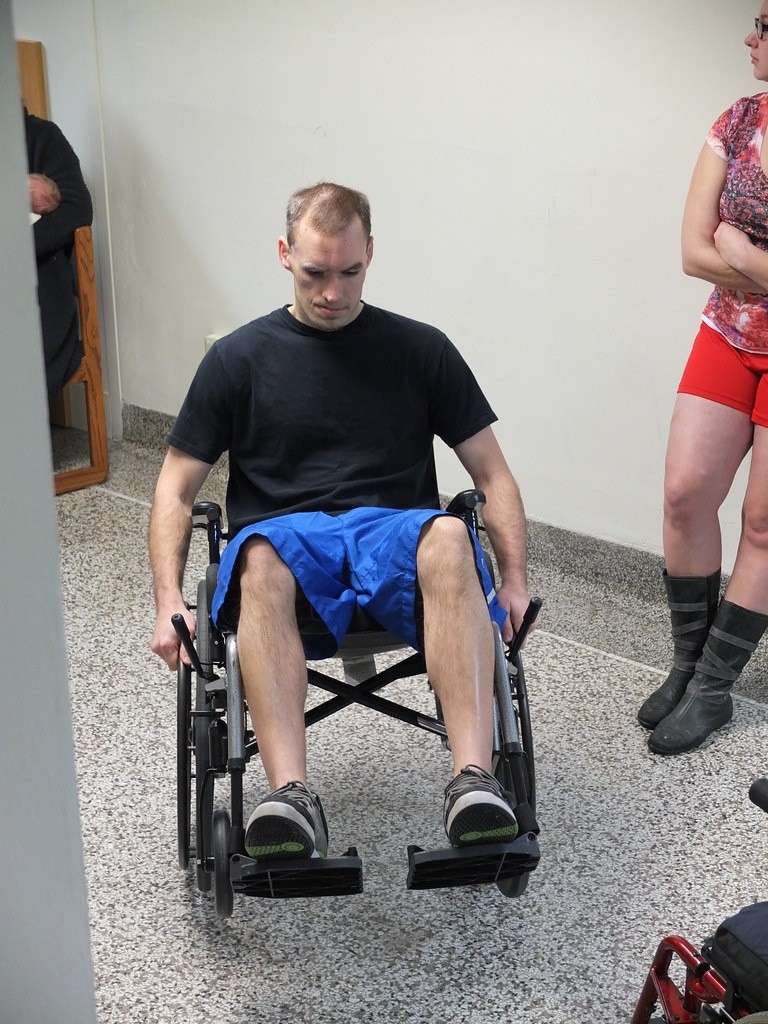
637 0 768 755
17 85 93 393
148 183 536 858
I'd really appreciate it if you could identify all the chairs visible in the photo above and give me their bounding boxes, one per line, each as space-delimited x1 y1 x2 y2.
52 225 110 498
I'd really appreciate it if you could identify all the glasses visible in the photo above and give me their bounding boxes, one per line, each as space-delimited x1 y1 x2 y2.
753 18 768 39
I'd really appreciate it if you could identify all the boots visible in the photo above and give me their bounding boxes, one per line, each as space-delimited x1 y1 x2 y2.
637 567 728 729
653 597 768 758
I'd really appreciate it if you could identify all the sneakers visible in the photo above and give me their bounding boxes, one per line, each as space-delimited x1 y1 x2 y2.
441 764 520 843
242 781 331 862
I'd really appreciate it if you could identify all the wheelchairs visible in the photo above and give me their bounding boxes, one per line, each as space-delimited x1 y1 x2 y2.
626 775 767 1024
175 489 544 919
